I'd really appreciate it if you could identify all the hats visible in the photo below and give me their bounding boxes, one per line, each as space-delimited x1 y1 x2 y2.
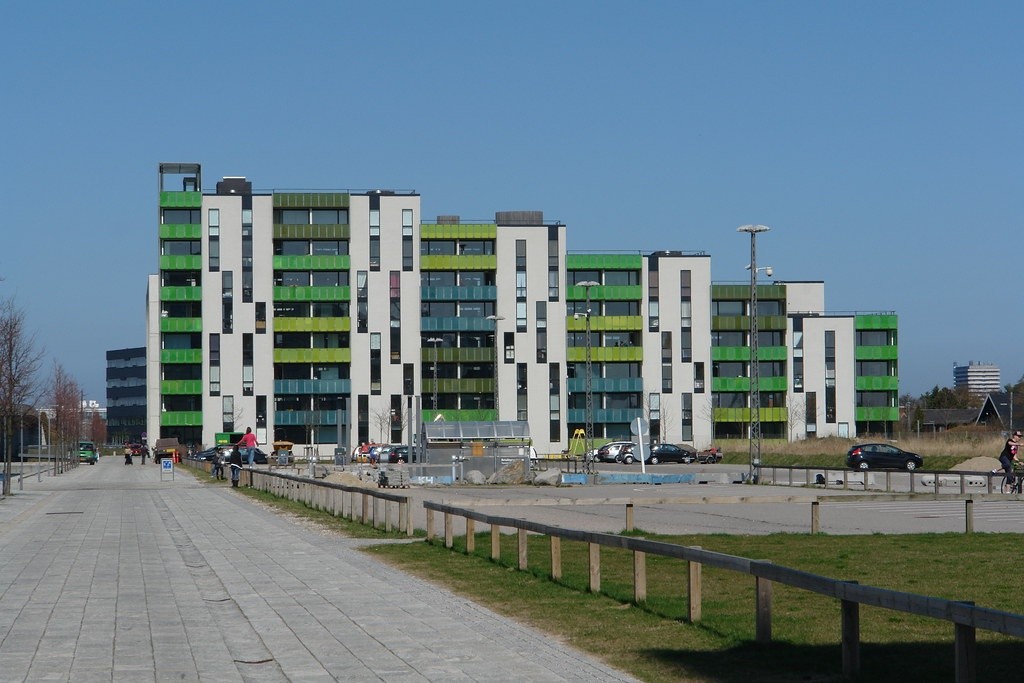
1013 431 1023 437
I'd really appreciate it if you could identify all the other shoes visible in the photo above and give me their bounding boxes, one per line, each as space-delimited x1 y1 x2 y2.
992 469 997 474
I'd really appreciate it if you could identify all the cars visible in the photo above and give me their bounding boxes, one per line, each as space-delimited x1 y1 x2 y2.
650 443 697 464
128 443 144 457
582 441 636 464
500 445 538 468
351 443 424 464
846 442 923 471
191 443 269 466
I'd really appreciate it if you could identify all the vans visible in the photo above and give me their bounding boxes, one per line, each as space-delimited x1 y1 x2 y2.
71 441 97 465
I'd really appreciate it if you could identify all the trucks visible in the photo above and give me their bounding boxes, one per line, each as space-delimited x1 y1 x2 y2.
152 438 183 465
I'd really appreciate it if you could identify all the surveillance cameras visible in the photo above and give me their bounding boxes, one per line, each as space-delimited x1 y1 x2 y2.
767 269 773 277
745 264 752 270
574 314 579 320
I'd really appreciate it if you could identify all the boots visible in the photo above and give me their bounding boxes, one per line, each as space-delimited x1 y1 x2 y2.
221 476 224 480
231 479 240 488
217 476 219 480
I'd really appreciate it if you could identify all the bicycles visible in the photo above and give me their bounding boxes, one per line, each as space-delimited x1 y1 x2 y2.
1000 462 1024 494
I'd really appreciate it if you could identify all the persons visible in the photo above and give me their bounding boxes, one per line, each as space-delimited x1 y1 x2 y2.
230 446 242 488
141 445 146 465
991 431 1024 494
361 438 375 451
238 427 259 468
215 448 226 480
125 446 130 460
710 445 717 453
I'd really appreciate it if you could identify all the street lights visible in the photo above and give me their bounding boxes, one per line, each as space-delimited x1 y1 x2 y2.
386 408 396 444
735 223 773 489
427 337 444 422
484 315 506 421
573 280 601 474
